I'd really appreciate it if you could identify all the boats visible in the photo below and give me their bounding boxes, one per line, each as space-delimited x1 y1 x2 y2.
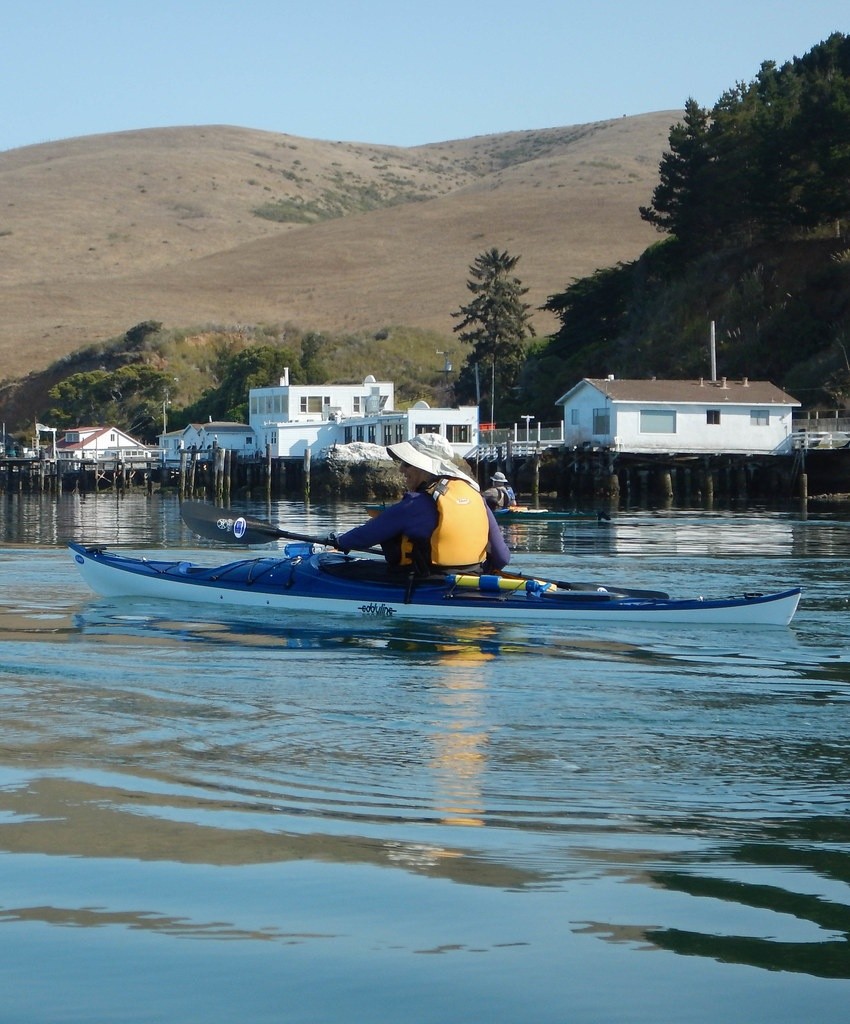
61 539 800 626
362 503 598 521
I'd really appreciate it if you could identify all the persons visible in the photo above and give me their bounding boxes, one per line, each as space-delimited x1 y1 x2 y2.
329 433 511 577
480 472 517 510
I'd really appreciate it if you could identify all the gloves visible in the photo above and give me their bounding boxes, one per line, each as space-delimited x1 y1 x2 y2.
328 531 350 555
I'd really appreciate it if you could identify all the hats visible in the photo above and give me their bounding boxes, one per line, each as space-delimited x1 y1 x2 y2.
489 471 508 483
386 432 481 492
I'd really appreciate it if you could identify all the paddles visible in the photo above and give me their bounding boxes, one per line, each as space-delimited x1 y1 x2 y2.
179 501 671 598
